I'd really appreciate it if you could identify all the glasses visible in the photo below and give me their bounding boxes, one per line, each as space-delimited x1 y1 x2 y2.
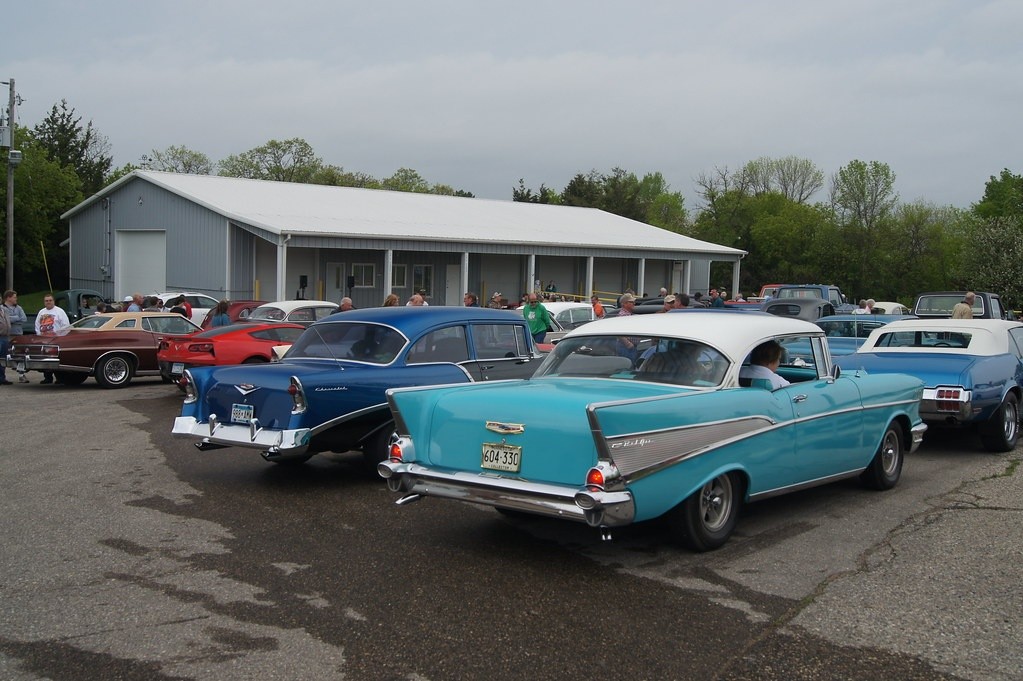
530 300 537 304
126 302 130 304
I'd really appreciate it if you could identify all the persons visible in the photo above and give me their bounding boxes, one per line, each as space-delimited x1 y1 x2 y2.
94 302 106 325
330 298 352 316
740 340 790 389
639 342 708 375
34 293 70 384
616 288 639 362
464 292 481 307
591 295 604 318
537 295 567 302
658 288 668 298
0 290 30 385
951 292 975 319
690 290 728 308
735 294 745 302
852 299 875 314
545 280 556 299
488 292 502 308
122 295 192 332
212 300 230 327
384 289 429 306
520 293 551 343
765 290 777 303
652 294 691 345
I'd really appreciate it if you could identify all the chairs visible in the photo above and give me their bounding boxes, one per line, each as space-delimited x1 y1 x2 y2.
640 353 698 386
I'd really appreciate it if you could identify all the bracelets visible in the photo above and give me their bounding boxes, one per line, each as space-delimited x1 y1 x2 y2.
625 342 630 345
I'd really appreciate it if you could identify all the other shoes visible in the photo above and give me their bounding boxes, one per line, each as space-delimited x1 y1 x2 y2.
18 376 30 383
2 380 13 385
39 375 53 383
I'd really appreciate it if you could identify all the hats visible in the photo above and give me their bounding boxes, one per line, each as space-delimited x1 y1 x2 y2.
123 296 134 301
620 293 636 303
721 292 727 296
663 294 676 303
494 292 501 298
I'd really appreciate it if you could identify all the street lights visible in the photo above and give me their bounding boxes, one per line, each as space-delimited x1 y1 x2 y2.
7 150 22 292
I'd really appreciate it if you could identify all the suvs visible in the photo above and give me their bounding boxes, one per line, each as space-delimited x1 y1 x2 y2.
144 290 219 329
912 291 1007 319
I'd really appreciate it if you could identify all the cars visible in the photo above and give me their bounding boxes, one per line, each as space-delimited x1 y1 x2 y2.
776 313 932 366
244 300 339 326
201 300 306 334
171 306 550 481
870 301 911 314
377 313 927 554
0 312 206 389
761 297 836 325
516 302 618 349
605 296 743 312
156 321 306 391
834 318 1023 454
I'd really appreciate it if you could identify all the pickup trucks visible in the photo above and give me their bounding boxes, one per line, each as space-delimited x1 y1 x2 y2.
22 289 104 336
724 283 846 311
747 284 781 303
